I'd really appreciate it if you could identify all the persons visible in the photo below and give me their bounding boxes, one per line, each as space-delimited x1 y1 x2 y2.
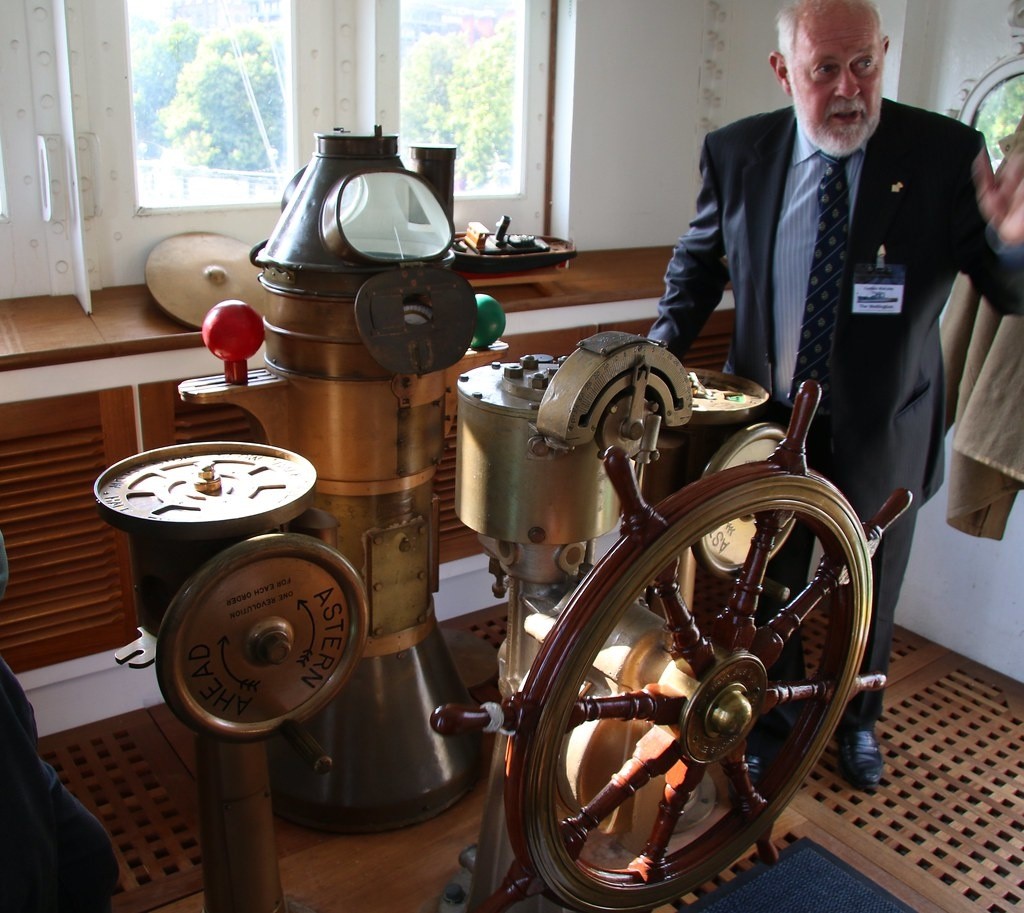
0 530 121 912
638 0 1024 793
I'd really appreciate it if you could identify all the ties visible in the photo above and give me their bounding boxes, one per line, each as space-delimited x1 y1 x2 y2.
789 151 855 415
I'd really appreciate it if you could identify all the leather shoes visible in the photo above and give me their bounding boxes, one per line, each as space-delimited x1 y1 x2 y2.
837 722 886 792
727 748 769 808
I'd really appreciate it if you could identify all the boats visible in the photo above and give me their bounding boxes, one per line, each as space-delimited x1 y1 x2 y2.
454 215 579 271
